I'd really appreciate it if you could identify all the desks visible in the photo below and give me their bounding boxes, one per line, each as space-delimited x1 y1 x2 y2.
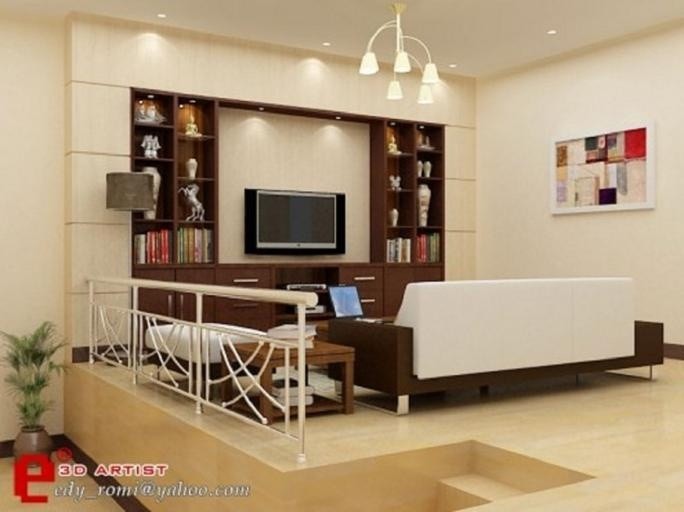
307 320 329 375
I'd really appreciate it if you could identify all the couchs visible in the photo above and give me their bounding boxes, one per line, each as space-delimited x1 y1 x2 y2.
327 276 663 416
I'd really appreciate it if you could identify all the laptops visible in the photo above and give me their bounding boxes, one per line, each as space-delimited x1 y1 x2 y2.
327 285 384 324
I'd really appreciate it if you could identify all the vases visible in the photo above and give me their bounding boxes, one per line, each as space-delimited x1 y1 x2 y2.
417 160 432 228
389 208 400 226
141 166 162 220
185 158 198 178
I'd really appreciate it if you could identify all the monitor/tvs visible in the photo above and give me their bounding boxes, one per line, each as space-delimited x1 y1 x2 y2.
256 190 338 249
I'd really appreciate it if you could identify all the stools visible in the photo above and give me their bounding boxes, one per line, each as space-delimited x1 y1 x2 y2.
144 323 276 401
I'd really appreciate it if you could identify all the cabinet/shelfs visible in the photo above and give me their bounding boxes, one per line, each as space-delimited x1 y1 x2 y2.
130 88 445 349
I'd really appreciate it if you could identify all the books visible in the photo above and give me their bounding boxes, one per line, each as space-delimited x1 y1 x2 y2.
271 377 315 409
556 128 645 210
133 225 213 265
266 322 318 350
386 232 440 264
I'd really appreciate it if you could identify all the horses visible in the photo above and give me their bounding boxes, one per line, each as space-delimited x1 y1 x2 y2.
177 184 205 222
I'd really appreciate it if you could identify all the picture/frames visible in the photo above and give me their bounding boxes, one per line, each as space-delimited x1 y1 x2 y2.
549 123 654 216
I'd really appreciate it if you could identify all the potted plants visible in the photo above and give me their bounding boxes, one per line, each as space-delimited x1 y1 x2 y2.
0 321 75 468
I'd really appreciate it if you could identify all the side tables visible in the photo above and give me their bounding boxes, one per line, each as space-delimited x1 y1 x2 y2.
220 341 356 426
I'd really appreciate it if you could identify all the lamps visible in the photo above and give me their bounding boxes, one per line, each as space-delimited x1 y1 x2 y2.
358 3 440 105
105 172 158 365
